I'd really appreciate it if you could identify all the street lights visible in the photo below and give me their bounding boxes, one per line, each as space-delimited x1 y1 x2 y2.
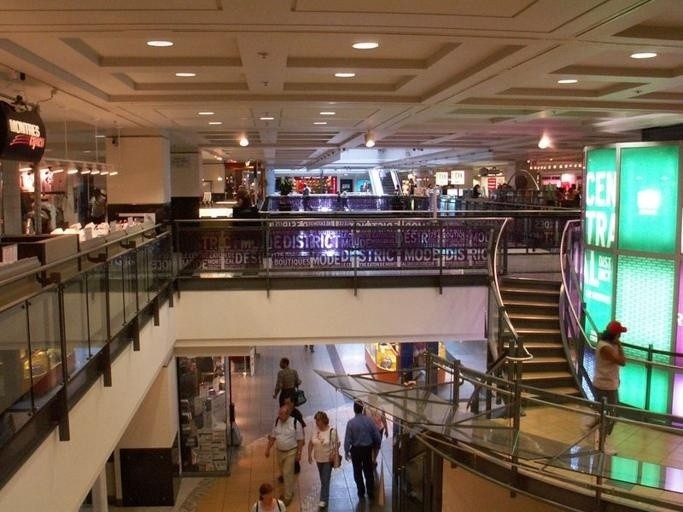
296 389 307 407
330 447 342 468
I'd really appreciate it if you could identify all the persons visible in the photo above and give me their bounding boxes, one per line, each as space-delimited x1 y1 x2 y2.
304 344 315 353
307 411 341 510
472 184 481 198
301 182 313 212
335 188 341 210
271 355 303 411
280 391 306 475
361 394 391 467
250 482 286 512
566 183 578 202
236 184 252 206
344 399 382 501
265 404 305 504
393 184 401 196
423 183 433 211
582 319 628 455
87 187 106 222
339 186 352 211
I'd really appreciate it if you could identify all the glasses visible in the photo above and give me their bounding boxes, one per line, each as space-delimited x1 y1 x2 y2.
362 127 376 148
16 103 123 180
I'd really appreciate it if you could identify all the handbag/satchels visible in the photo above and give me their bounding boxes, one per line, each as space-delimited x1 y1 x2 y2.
297 451 302 455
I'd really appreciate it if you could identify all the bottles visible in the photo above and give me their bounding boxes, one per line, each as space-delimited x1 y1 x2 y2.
607 320 627 334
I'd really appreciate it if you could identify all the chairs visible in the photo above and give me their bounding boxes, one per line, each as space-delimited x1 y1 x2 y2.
357 462 377 500
318 501 328 508
280 492 293 507
